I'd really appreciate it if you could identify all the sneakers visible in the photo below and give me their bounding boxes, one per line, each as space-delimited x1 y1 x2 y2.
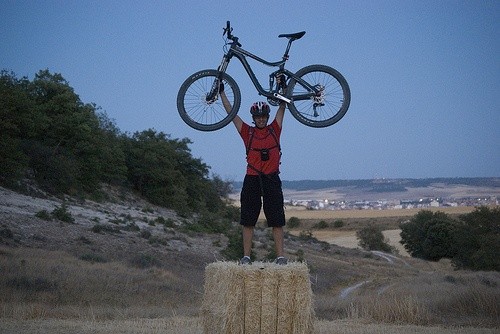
275 256 287 265
238 257 252 265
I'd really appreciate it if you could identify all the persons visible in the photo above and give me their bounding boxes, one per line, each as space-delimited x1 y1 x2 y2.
219 74 288 265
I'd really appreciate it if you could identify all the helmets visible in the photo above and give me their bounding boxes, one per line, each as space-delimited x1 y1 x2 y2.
250 102 270 114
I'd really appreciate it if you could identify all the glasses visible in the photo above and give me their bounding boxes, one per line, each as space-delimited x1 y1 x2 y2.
253 115 269 119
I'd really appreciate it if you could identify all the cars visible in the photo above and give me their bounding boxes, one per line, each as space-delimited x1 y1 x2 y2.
306 200 320 210
288 200 297 206
297 201 307 207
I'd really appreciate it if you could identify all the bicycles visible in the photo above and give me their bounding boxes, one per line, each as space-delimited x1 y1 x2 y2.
177 21 351 132
